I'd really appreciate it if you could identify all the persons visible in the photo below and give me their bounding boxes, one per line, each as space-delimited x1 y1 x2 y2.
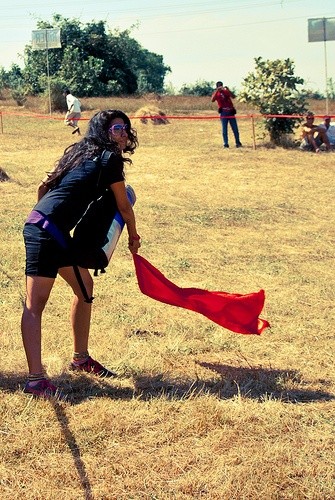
62 87 83 137
20 109 142 403
294 111 335 155
210 81 244 150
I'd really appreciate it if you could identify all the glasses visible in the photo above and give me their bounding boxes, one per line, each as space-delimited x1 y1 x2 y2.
109 124 128 136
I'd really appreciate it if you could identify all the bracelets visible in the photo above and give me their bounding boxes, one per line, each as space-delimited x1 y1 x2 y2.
129 233 141 241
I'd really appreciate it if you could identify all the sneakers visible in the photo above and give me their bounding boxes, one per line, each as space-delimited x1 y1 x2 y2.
69 356 115 377
24 378 68 401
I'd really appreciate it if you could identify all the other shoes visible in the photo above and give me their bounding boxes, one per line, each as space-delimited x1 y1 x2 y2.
236 143 241 148
72 127 79 134
224 144 229 147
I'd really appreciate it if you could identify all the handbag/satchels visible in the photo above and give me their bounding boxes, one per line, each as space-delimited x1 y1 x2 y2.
71 185 137 303
